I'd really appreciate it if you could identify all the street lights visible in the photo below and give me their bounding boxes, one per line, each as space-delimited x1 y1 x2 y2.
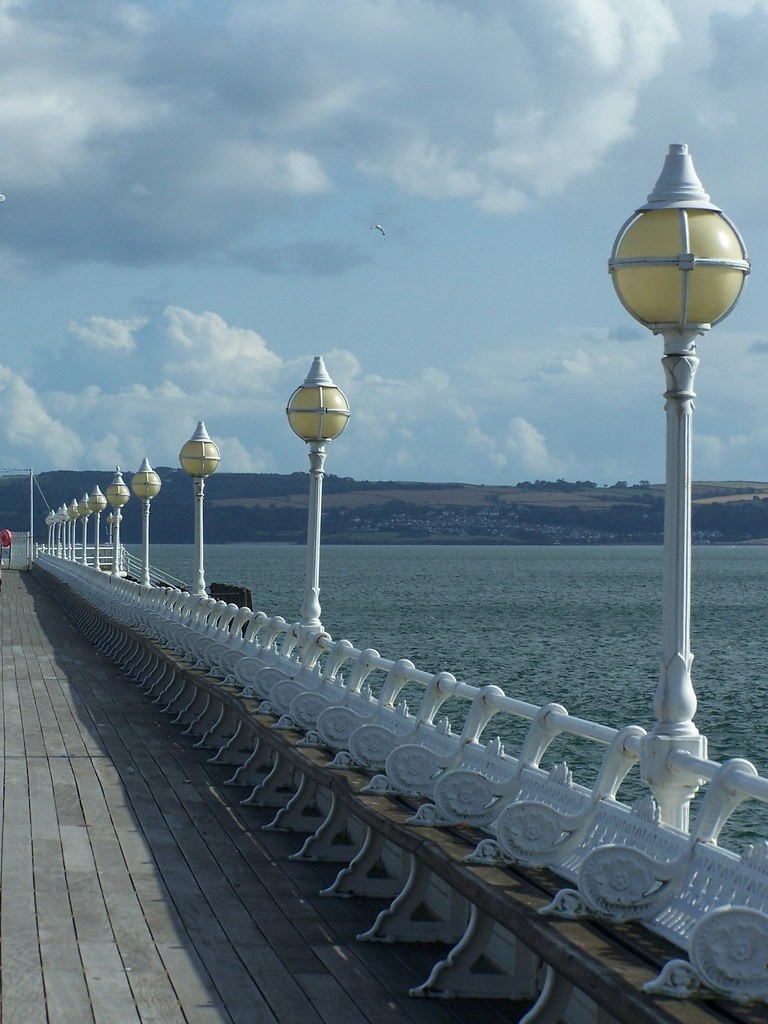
68 498 80 564
607 140 751 838
78 493 93 566
106 466 129 584
130 457 161 589
285 355 349 638
178 419 224 606
45 503 69 560
88 485 107 572
